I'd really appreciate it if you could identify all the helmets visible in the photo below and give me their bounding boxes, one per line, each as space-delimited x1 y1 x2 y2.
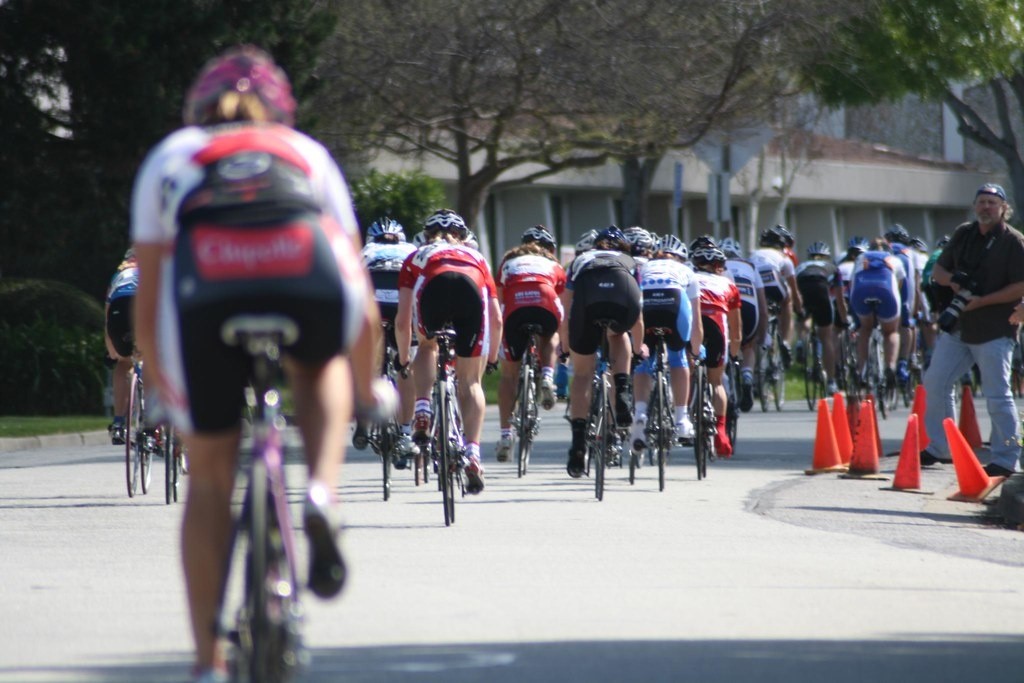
366 204 956 264
180 52 294 128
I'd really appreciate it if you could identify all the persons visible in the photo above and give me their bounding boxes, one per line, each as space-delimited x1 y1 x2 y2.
129 49 382 683
495 223 950 477
366 207 502 494
919 184 1024 478
104 249 134 445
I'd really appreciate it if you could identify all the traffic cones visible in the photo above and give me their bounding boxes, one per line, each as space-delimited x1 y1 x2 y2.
839 398 891 482
941 416 1008 503
902 386 930 452
845 392 884 458
958 384 986 450
803 399 849 475
879 413 938 495
829 388 852 469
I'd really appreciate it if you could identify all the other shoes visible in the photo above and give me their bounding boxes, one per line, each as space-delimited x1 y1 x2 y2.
305 491 346 601
918 445 955 468
147 438 157 452
107 426 123 446
984 464 1012 478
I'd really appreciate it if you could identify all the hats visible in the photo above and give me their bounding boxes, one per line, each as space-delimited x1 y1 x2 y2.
976 182 1006 201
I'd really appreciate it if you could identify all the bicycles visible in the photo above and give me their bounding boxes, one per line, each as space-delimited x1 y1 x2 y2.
357 293 942 532
151 407 193 504
209 314 315 683
108 326 163 496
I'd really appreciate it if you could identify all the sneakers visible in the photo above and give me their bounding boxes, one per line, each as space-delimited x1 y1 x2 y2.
380 366 911 493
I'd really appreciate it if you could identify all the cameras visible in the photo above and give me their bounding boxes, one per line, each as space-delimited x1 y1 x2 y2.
937 272 979 334
